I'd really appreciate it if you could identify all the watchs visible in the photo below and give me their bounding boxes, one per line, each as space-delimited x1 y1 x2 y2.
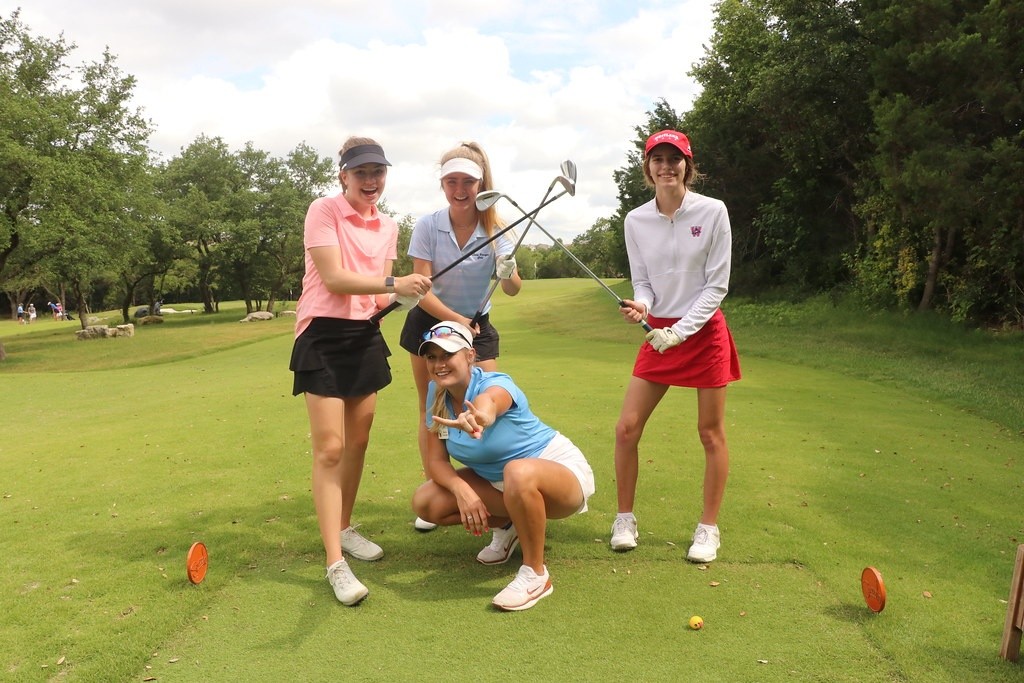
385 276 394 293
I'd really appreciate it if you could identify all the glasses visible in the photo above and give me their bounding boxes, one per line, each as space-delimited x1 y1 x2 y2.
421 326 471 347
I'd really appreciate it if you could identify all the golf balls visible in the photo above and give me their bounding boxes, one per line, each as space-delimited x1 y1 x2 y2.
690 616 704 630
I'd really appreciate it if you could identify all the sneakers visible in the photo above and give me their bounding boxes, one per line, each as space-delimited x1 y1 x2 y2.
687 522 720 563
340 523 384 560
492 565 554 611
325 556 369 606
476 523 520 565
610 512 639 550
414 517 437 531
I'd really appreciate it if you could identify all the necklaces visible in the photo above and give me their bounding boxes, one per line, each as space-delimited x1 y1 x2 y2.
451 215 476 229
453 406 460 415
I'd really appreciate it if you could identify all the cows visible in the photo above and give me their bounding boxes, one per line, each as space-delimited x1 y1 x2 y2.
134 299 165 318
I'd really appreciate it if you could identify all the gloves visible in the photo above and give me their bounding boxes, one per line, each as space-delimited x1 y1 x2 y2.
646 327 681 354
495 254 516 279
389 293 425 312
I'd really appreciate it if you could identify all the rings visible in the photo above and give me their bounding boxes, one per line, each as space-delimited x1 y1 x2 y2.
468 430 475 434
466 516 473 520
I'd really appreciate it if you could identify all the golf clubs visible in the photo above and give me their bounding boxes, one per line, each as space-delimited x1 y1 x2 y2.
368 156 578 325
469 174 576 330
475 189 653 333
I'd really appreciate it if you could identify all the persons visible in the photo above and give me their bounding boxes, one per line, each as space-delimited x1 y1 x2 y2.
48 302 63 321
289 137 433 606
411 320 595 611
611 130 742 562
155 299 161 315
400 142 522 532
17 303 36 324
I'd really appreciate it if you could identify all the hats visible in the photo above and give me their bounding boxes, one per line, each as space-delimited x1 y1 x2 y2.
439 158 484 180
645 130 692 159
340 145 392 170
418 321 473 357
